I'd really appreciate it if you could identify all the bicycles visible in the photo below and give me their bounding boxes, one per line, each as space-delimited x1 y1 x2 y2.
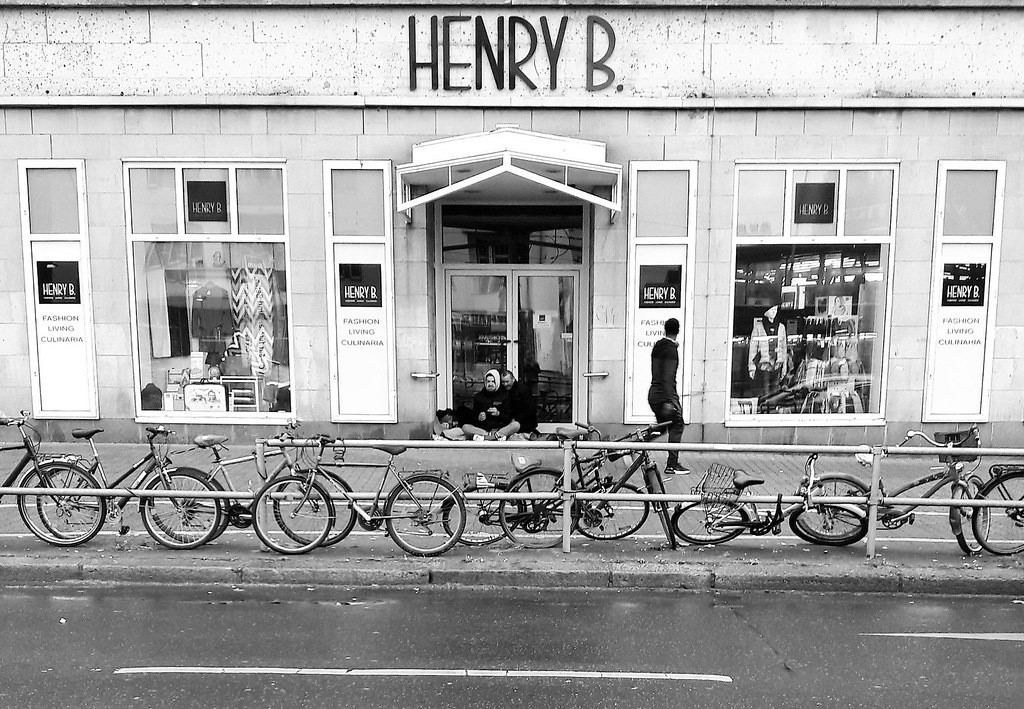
434 422 650 548
274 433 467 557
972 462 1024 556
33 426 221 551
669 452 867 548
793 423 991 556
148 417 339 556
1 409 107 548
502 419 679 552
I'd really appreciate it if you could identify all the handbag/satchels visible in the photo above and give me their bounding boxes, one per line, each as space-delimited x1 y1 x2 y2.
183 378 229 412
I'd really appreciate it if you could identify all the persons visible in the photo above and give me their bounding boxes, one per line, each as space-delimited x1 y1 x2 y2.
635 318 692 475
751 297 788 414
473 370 538 441
523 351 541 396
462 369 520 441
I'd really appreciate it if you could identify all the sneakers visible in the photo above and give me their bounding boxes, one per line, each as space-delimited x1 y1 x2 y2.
664 463 691 475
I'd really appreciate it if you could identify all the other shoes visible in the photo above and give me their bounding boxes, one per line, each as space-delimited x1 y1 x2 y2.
472 434 484 440
525 432 535 439
497 436 507 441
